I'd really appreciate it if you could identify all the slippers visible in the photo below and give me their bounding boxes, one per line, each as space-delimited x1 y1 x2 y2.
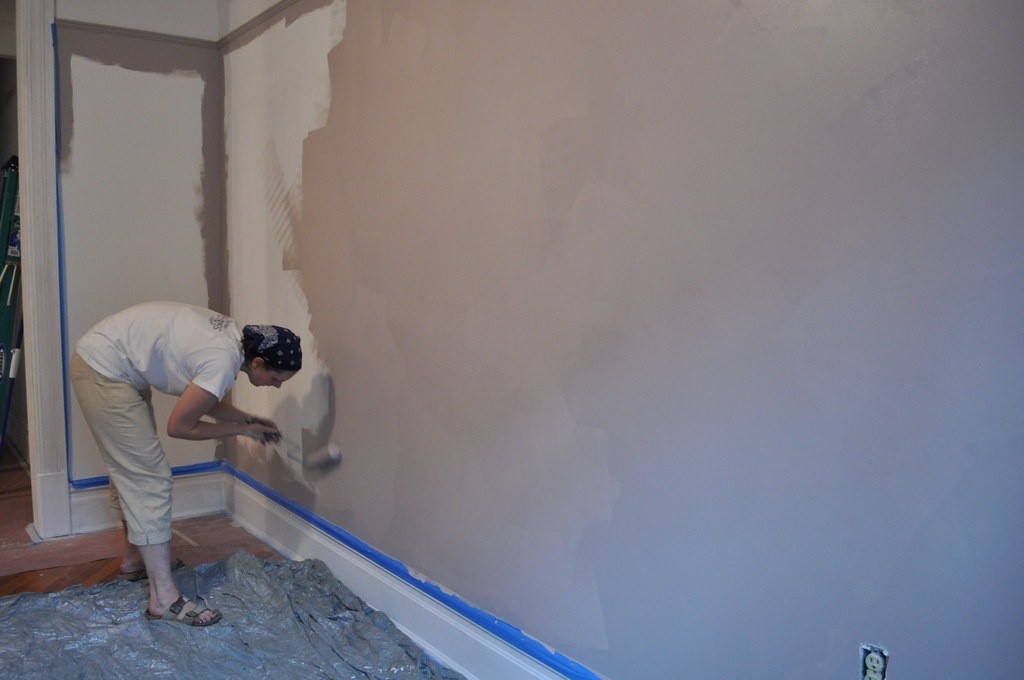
116 558 222 625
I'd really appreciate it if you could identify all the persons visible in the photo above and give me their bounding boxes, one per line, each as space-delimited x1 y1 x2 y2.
69 300 305 627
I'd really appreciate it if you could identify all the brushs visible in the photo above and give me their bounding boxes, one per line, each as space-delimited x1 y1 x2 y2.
246 415 341 471
